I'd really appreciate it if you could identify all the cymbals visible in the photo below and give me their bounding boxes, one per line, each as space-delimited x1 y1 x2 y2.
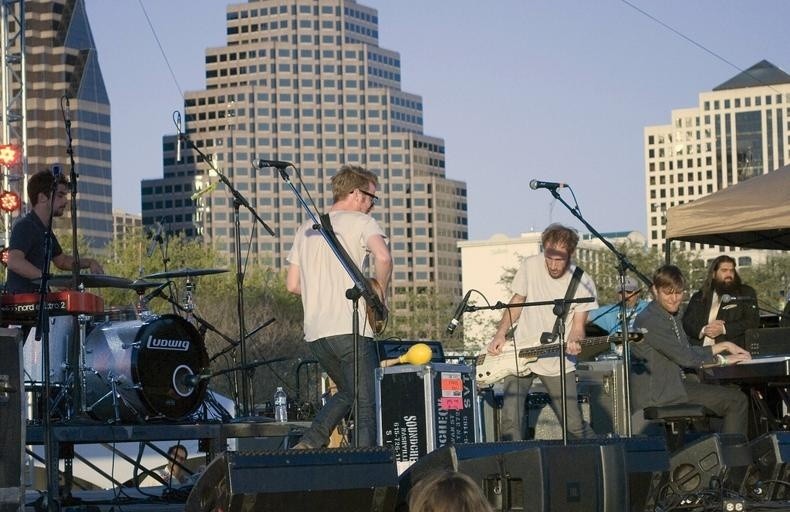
144 267 229 278
127 280 164 288
31 273 132 288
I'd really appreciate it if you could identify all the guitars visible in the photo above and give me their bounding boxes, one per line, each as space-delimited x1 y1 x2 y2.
475 327 645 387
364 277 387 335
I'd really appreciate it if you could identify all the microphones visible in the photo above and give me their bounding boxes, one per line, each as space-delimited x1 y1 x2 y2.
722 294 756 303
141 282 169 305
66 101 71 128
530 180 568 190
52 166 61 178
148 217 165 257
253 158 292 169
176 113 181 161
448 292 471 335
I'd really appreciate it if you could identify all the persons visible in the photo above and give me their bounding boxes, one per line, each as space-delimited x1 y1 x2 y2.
593 276 652 336
408 471 494 511
6 167 105 294
487 223 599 440
285 164 393 448
680 254 759 355
628 264 751 440
138 445 188 487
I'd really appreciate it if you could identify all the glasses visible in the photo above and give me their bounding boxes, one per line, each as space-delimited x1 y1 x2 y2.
349 188 379 205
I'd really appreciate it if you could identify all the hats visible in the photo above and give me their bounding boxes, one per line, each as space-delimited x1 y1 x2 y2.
618 279 639 293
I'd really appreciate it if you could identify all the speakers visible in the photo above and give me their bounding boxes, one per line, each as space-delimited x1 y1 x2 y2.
643 434 755 512
750 430 790 500
185 446 400 512
398 435 670 512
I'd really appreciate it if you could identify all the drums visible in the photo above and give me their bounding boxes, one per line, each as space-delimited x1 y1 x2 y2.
8 314 79 390
78 309 211 425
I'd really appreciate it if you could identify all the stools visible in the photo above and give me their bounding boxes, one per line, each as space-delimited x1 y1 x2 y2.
644 404 717 454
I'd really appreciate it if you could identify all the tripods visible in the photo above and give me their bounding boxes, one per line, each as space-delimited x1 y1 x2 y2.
49 316 147 425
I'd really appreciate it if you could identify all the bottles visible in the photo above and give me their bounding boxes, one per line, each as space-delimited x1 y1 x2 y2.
273 385 289 424
776 291 787 320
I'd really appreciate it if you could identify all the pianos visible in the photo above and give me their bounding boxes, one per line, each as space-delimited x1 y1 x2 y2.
696 350 790 383
0 289 104 326
